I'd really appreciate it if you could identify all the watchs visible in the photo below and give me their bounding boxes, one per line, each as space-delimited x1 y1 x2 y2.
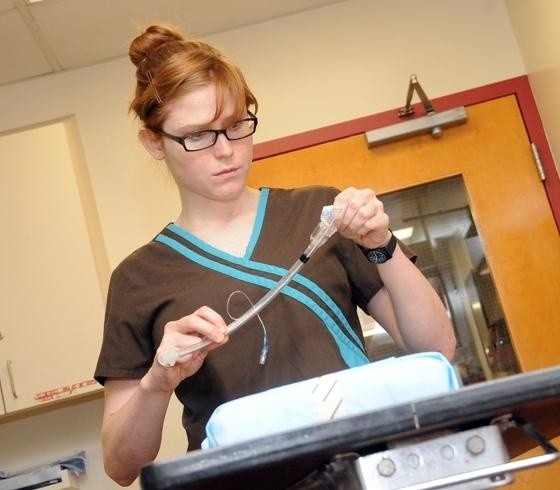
356 228 397 265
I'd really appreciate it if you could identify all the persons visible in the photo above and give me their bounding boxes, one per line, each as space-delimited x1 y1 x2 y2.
94 25 456 490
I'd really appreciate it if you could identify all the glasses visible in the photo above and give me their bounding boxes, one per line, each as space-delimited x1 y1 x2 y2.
145 110 258 153
